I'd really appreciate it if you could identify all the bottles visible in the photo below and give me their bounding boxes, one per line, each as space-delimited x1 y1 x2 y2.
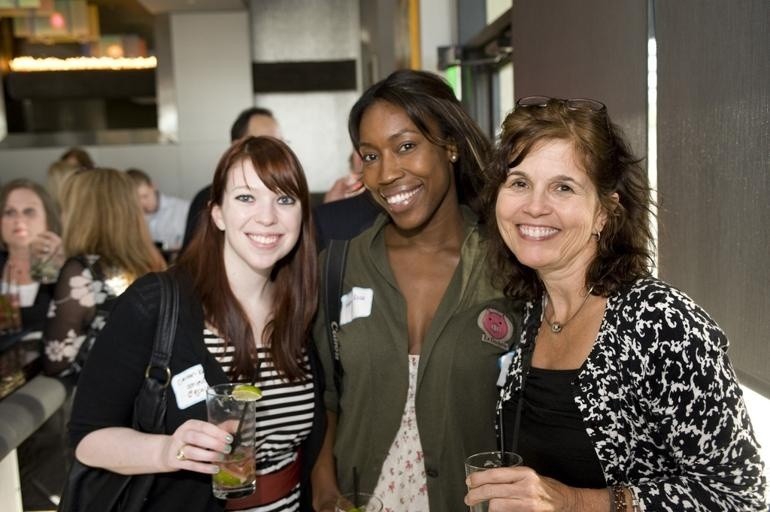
1 262 30 394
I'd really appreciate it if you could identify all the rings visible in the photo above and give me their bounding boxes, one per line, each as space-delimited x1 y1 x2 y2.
176 450 185 460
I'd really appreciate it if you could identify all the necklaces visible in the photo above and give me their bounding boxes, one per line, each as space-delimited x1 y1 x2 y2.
541 285 595 333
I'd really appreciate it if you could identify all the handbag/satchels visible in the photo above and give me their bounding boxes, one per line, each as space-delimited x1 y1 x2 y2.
58 275 180 512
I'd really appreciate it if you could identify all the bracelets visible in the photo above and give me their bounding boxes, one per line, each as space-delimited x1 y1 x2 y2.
607 482 627 511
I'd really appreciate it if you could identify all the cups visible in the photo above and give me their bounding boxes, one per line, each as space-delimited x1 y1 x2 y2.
206 383 259 500
31 233 65 282
335 492 385 512
463 448 528 512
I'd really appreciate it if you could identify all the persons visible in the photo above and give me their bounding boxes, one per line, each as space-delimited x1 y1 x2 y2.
127 169 192 249
180 108 288 251
466 94 767 510
63 139 321 510
2 150 165 512
312 71 527 512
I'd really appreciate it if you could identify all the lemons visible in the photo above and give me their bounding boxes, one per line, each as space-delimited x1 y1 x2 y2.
232 386 263 402
213 467 247 487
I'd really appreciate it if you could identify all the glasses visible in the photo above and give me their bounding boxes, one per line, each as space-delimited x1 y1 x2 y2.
514 96 608 114
